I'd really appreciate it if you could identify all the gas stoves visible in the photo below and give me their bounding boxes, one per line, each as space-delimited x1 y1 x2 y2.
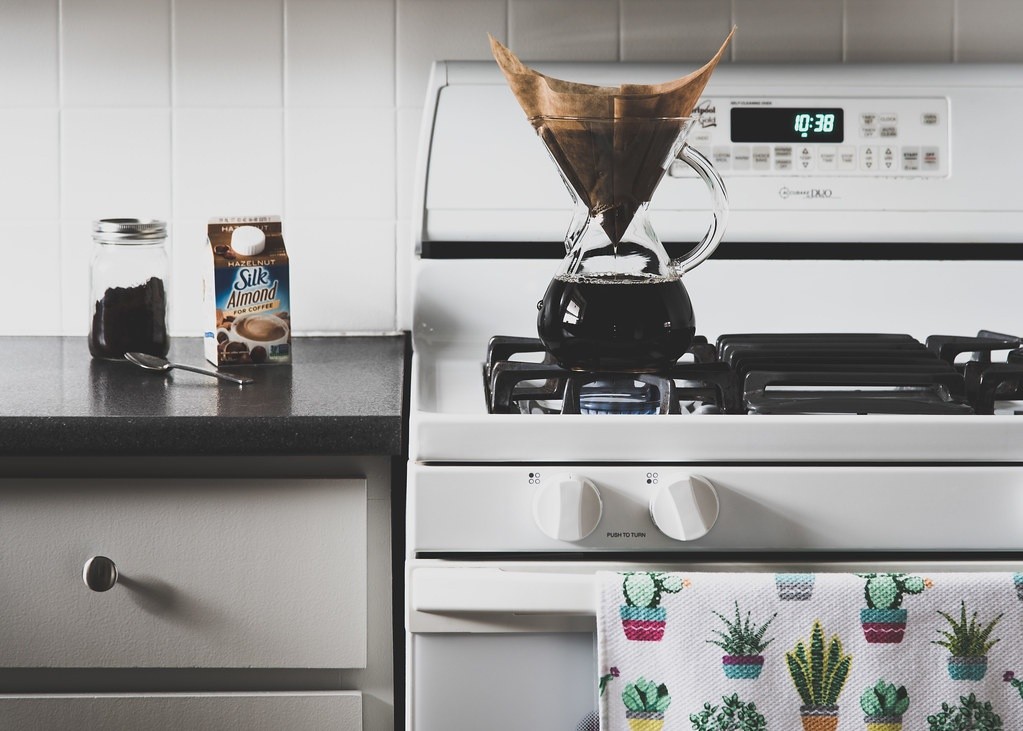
404 61 1023 559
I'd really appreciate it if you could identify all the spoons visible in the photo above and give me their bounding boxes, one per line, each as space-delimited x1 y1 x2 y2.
124 352 254 385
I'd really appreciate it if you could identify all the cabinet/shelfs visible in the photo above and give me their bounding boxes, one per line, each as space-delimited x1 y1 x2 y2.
0 335 408 731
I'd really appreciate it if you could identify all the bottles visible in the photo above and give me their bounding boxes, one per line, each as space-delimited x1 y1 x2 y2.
87 220 169 360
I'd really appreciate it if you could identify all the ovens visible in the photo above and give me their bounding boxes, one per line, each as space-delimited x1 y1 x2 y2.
404 556 1023 731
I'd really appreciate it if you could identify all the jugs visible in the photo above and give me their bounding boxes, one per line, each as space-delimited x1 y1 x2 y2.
528 114 729 374
